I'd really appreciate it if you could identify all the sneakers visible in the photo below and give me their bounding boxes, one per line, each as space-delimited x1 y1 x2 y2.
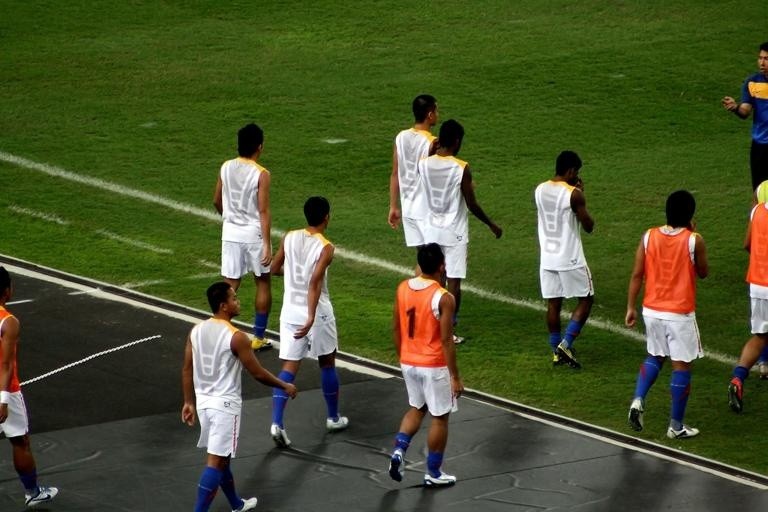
389 450 404 481
729 378 744 415
667 425 699 440
553 346 580 368
232 496 257 512
24 486 57 506
250 336 272 349
327 417 350 430
629 401 646 432
270 423 292 450
425 472 457 487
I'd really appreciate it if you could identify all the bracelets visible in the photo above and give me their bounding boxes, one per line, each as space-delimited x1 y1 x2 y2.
734 103 740 115
1 391 10 404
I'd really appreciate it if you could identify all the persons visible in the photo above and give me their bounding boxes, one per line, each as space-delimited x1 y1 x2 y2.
180 281 296 511
388 95 443 283
624 190 708 438
723 41 768 192
213 123 272 350
535 151 594 369
389 242 463 486
0 265 59 508
727 179 768 416
418 117 502 344
270 196 349 448
